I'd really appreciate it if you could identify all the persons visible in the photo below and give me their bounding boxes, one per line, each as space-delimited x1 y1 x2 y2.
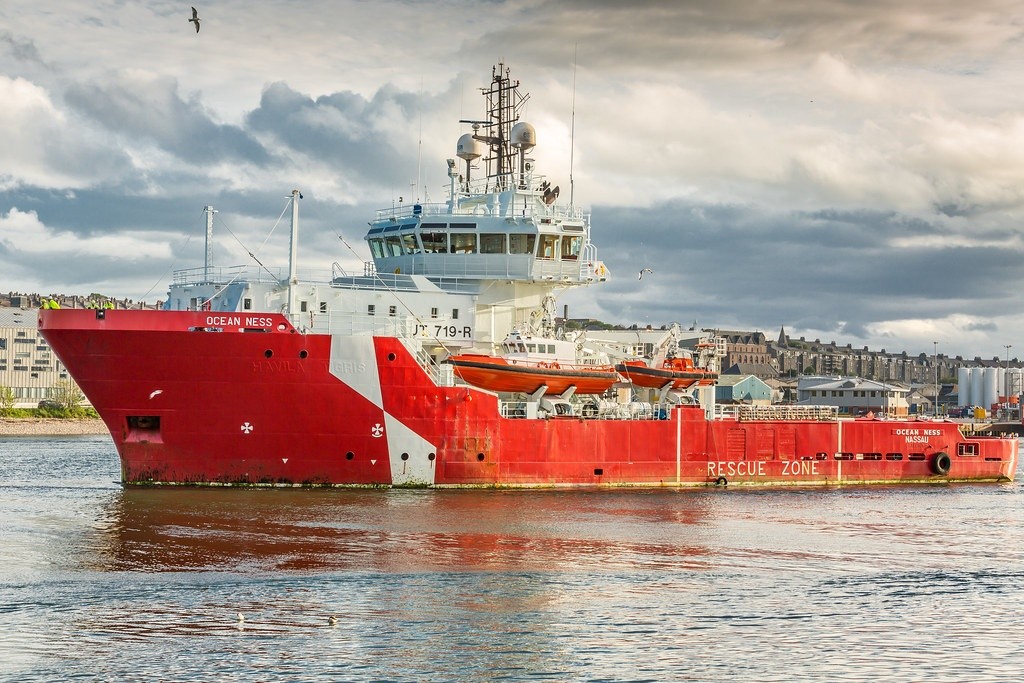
432 247 437 253
47 296 60 309
86 300 100 309
41 299 49 309
104 299 114 309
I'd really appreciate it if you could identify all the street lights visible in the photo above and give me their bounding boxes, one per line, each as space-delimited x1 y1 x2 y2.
933 341 939 419
1003 345 1012 421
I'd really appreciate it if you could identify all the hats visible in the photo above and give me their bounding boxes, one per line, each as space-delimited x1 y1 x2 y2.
46 296 52 300
107 299 111 301
41 299 46 302
90 300 95 303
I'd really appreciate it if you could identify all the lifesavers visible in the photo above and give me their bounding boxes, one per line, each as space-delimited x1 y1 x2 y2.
549 362 561 369
537 361 548 368
717 477 727 485
932 452 952 475
582 401 598 420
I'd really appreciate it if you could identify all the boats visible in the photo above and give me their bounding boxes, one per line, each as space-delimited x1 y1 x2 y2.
614 327 720 390
447 319 620 395
35 63 1017 490
